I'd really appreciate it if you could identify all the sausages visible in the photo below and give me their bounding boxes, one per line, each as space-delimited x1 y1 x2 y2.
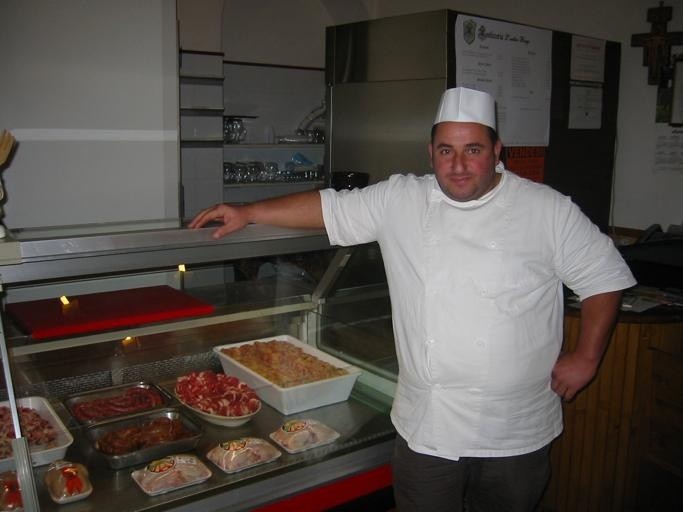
72 387 163 422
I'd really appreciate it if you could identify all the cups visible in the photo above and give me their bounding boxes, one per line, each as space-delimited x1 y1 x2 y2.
223 161 278 182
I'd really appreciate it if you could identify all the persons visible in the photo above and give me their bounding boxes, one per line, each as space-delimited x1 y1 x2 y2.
186 86 638 511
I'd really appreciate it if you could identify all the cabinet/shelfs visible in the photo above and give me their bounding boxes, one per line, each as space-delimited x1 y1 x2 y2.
0 221 399 512
224 142 326 187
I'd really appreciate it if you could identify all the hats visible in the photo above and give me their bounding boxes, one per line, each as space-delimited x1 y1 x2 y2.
433 86 496 132
